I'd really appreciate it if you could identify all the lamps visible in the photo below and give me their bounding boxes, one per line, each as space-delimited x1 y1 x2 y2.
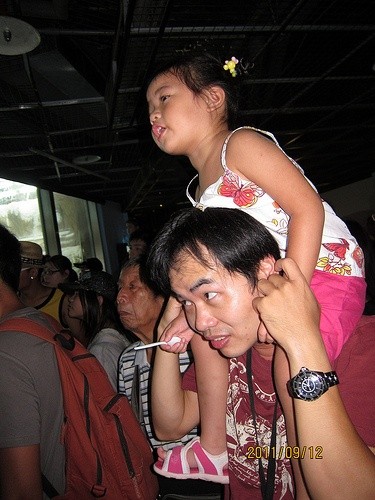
0 15 41 56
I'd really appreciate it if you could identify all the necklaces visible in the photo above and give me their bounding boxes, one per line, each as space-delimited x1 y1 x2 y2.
247 347 279 500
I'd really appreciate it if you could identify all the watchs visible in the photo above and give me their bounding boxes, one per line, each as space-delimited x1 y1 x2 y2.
287 367 339 402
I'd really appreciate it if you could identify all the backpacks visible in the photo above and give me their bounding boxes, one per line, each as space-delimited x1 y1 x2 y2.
0 312 159 500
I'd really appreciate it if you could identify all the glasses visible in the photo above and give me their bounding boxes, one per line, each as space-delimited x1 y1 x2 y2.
43 269 64 276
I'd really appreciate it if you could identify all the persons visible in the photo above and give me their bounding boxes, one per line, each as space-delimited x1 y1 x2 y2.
18 240 104 351
0 223 74 500
146 207 375 500
117 255 225 500
61 272 132 394
146 51 366 484
121 218 155 263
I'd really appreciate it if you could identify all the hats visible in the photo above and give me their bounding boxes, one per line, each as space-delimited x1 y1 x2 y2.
57 271 119 301
19 241 48 268
74 258 103 271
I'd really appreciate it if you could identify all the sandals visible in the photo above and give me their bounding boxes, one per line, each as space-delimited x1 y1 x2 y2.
152 436 230 484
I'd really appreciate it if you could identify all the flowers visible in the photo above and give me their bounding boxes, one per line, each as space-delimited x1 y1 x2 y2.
222 52 242 78
